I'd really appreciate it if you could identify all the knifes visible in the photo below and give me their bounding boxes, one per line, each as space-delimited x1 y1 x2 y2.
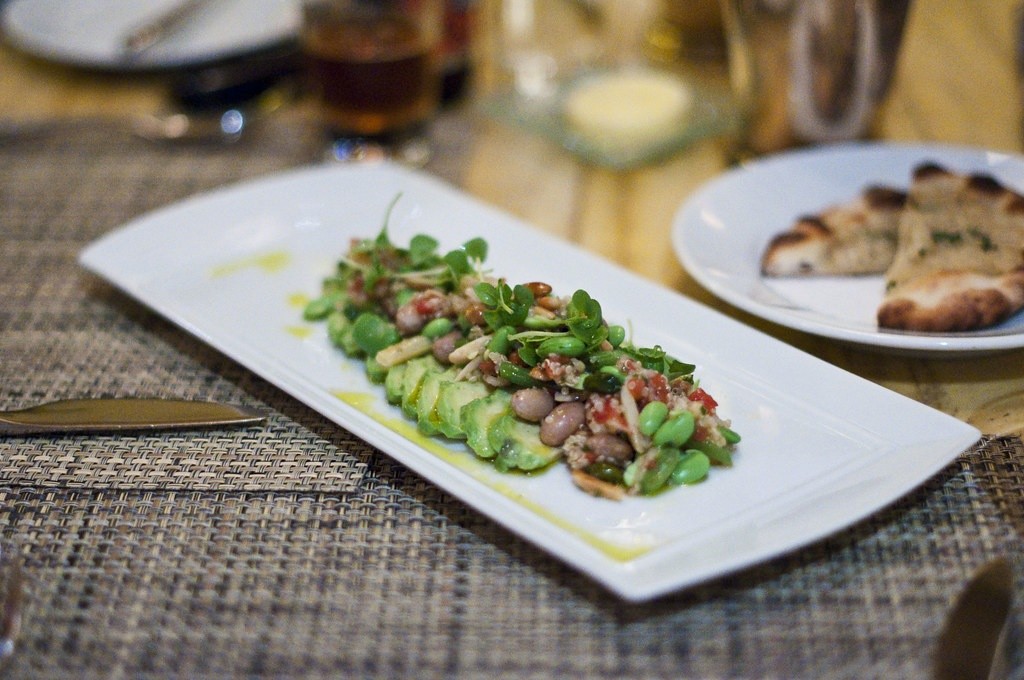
0 398 269 433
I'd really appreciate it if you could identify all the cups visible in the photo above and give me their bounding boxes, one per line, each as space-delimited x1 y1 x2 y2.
299 0 442 148
719 0 909 152
438 1 475 112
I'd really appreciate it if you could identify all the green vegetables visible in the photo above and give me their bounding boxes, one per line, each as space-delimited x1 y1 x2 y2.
326 193 696 391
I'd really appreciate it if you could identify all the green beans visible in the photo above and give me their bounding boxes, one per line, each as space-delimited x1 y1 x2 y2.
639 400 741 492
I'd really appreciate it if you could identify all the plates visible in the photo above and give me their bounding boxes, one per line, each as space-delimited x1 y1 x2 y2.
0 0 303 69
78 153 981 605
481 51 765 180
667 142 1024 359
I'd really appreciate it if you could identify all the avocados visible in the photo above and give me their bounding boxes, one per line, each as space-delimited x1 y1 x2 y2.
306 296 561 470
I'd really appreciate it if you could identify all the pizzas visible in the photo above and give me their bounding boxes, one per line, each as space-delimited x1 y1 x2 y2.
762 161 1023 330
